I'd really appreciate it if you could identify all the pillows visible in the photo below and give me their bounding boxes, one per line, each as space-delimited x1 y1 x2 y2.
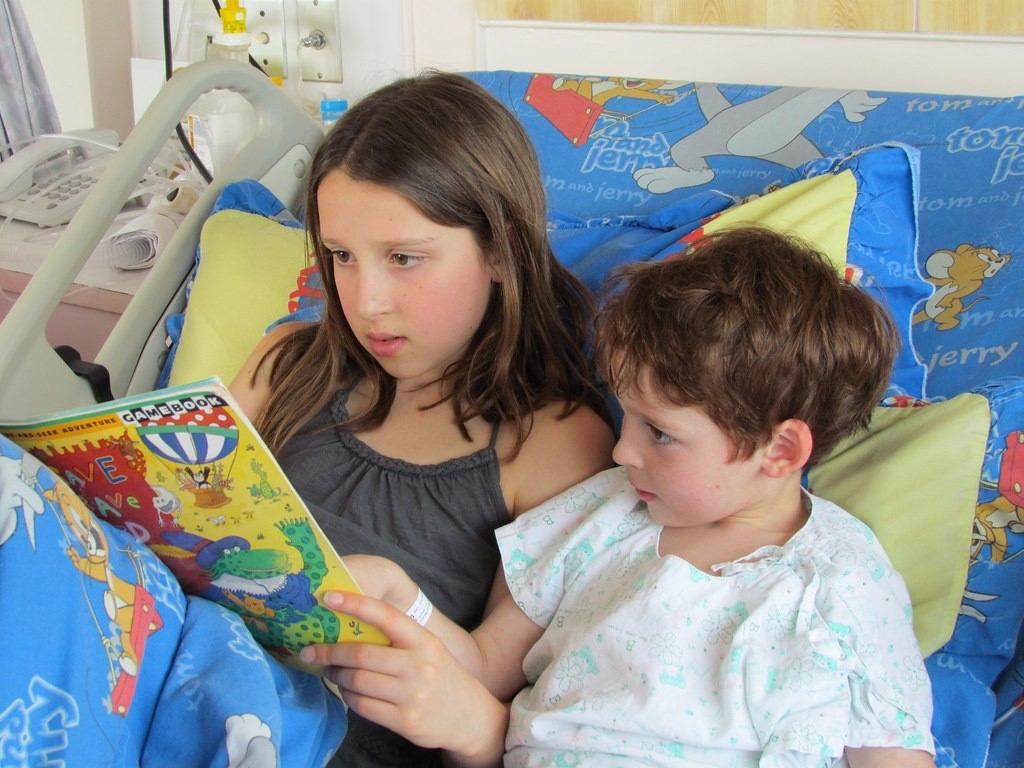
154 141 936 408
800 378 1024 768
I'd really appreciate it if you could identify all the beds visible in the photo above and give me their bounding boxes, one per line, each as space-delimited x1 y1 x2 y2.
0 18 1024 768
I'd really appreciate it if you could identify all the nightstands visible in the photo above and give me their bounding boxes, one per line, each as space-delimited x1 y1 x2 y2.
0 205 162 364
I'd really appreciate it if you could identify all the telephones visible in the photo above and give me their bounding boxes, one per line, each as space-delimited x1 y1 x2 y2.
0 127 156 228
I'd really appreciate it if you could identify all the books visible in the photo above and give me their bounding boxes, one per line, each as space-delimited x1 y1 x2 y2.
0 376 391 676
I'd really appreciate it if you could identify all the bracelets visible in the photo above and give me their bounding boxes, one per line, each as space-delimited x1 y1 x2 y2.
406 589 432 626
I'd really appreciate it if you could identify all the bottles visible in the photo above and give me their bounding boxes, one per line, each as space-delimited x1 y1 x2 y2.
199 33 258 178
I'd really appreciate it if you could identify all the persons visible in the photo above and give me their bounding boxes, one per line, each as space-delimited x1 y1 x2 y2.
339 225 936 767
229 71 615 768
